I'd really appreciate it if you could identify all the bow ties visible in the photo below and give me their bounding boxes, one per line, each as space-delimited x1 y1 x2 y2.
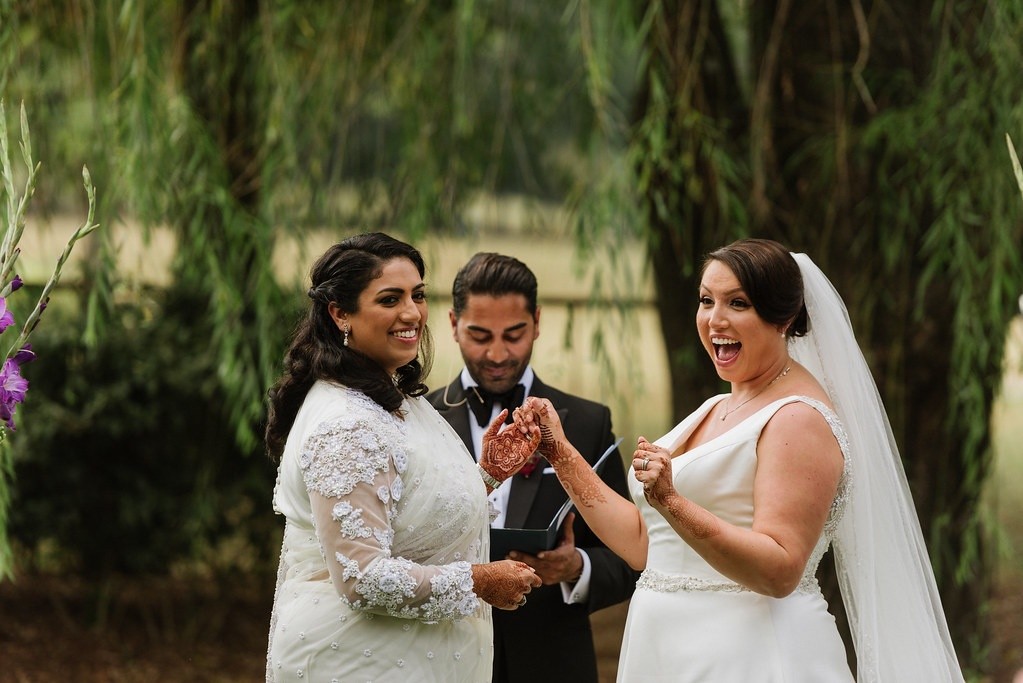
465 386 528 431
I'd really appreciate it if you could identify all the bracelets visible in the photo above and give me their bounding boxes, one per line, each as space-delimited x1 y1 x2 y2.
473 462 502 490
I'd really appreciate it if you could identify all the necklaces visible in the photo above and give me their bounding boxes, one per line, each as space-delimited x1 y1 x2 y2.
720 360 795 421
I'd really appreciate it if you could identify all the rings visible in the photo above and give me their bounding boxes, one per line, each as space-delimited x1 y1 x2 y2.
516 595 526 606
513 406 522 412
523 432 532 441
641 457 650 471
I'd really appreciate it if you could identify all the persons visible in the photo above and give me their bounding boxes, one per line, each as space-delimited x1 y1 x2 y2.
263 232 541 683
422 250 644 683
515 237 861 682
